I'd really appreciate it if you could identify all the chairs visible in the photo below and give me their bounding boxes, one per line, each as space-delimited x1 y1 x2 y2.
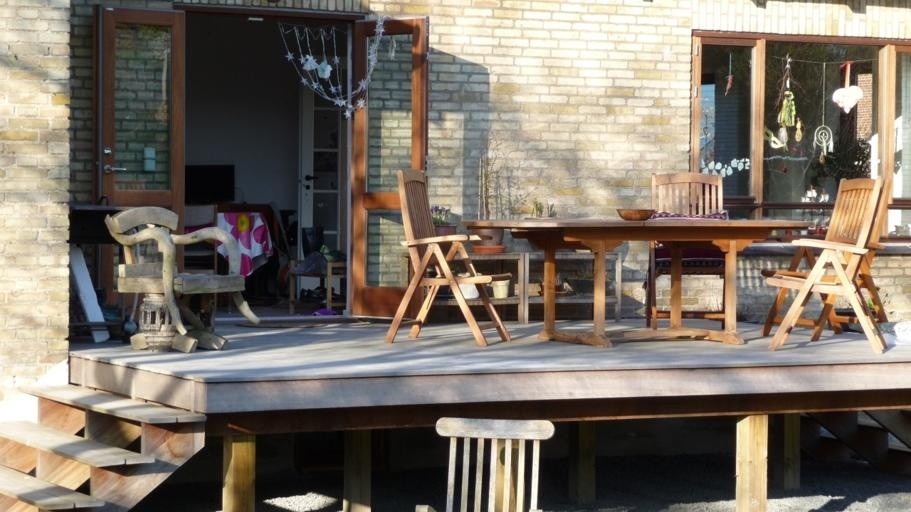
432 417 555 512
105 207 261 354
647 175 730 327
803 178 892 330
387 170 514 342
761 180 886 354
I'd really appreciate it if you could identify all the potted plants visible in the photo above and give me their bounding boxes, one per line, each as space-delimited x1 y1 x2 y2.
430 205 460 249
526 200 563 248
467 170 506 254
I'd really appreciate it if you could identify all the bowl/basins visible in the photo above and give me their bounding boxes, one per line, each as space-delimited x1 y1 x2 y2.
616 207 655 221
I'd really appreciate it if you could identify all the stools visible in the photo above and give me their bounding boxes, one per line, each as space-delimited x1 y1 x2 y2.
289 257 346 314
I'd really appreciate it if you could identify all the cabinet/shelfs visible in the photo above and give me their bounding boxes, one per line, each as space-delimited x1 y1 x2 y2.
735 243 824 257
402 251 623 324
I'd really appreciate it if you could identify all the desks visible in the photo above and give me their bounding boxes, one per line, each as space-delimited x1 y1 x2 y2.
465 215 815 345
